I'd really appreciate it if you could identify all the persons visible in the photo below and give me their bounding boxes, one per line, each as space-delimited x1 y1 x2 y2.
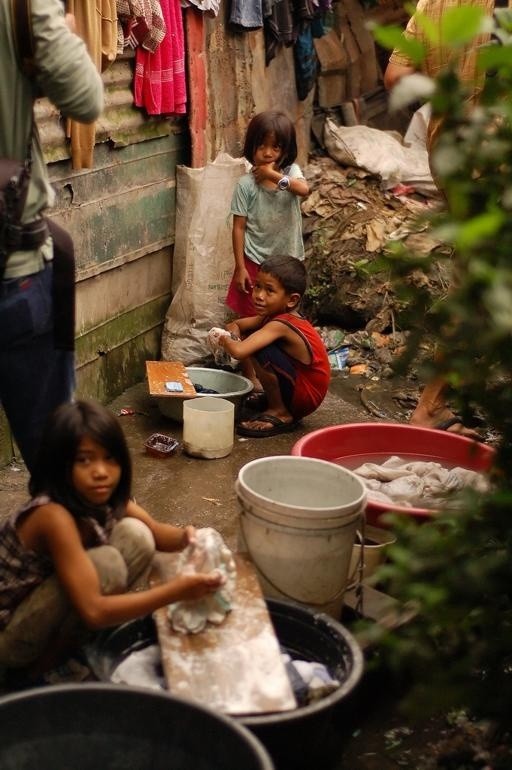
384 0 512 440
0 401 222 697
0 0 103 497
207 255 330 430
225 111 309 401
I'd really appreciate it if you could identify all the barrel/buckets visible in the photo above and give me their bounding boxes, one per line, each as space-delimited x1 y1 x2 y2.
0 682 277 770
182 395 235 458
234 455 369 620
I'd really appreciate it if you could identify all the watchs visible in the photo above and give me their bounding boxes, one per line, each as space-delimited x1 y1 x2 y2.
278 175 290 191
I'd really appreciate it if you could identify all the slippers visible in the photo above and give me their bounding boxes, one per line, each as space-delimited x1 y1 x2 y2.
236 413 298 438
